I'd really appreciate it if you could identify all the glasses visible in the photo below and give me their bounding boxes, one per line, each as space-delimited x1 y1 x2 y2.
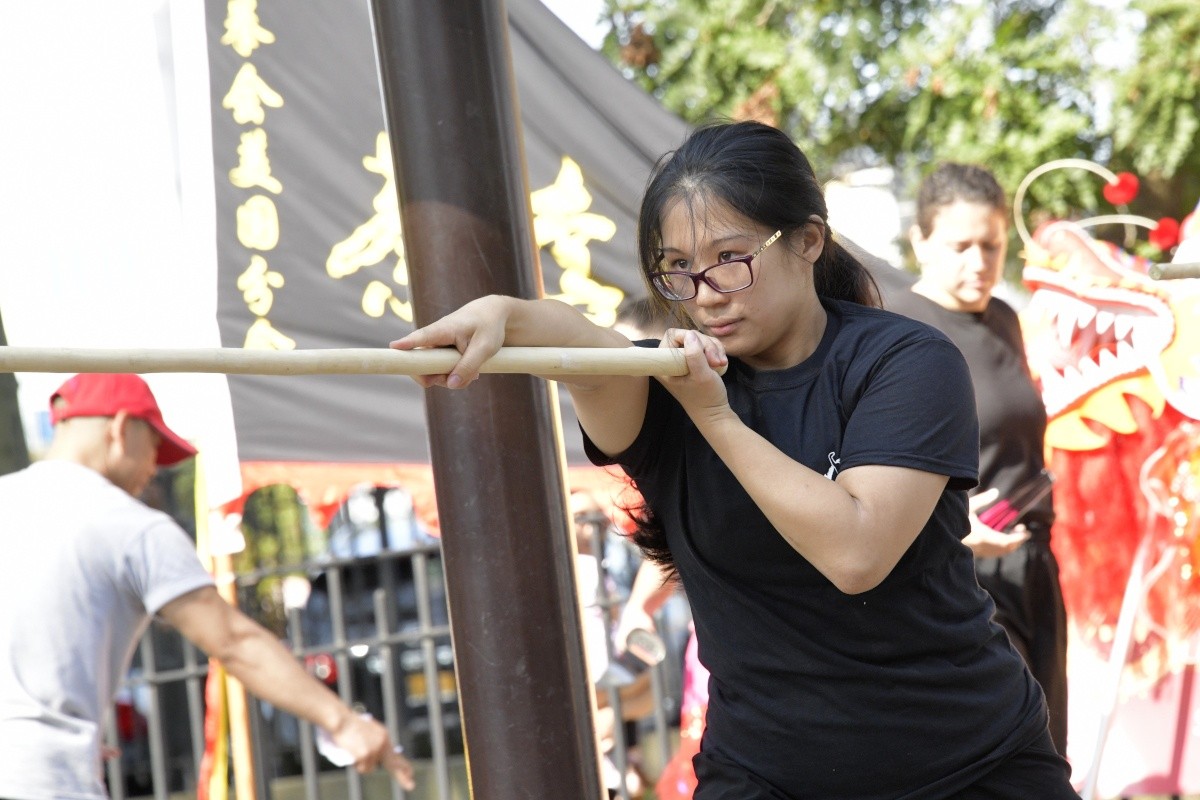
648 228 786 303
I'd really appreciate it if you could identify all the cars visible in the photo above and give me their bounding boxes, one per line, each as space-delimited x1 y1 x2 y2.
103 615 214 800
266 542 468 781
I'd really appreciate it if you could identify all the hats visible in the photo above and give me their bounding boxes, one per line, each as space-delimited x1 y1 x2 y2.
48 372 198 472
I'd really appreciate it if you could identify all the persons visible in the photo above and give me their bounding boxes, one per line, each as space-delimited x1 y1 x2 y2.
878 162 1066 754
612 547 709 800
0 373 414 800
389 119 1083 800
